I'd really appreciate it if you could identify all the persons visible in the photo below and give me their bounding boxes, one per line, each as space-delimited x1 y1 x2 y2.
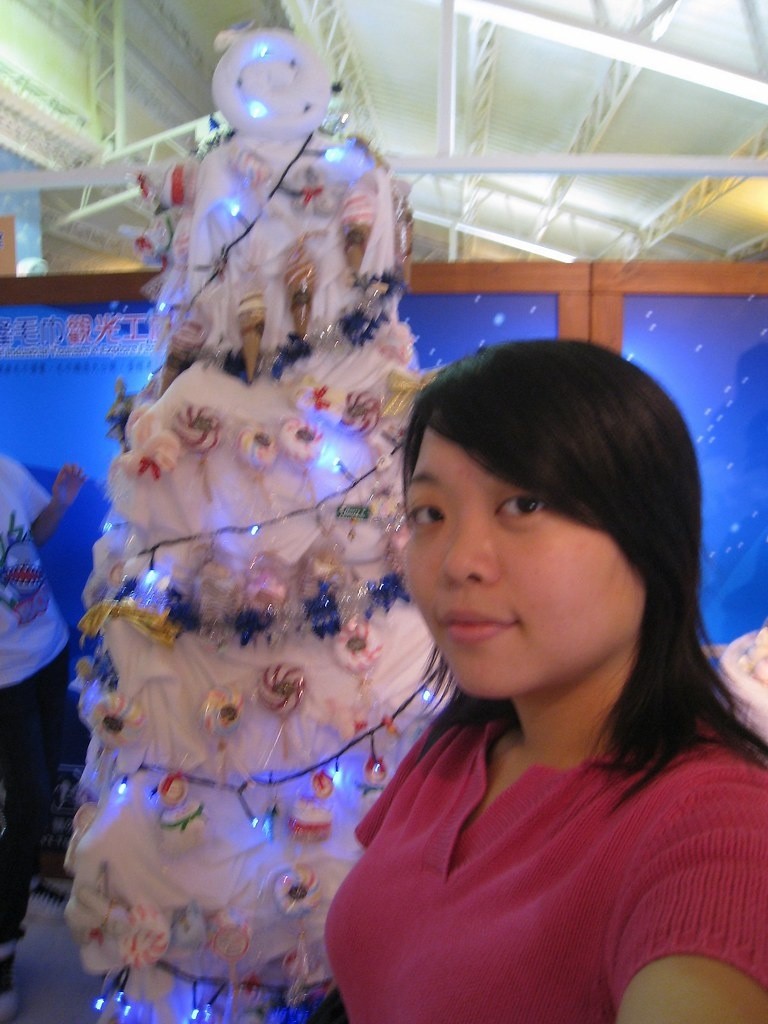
0 454 86 1022
322 340 766 1024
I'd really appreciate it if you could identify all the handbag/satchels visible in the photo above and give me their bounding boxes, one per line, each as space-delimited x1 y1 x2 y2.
306 990 348 1024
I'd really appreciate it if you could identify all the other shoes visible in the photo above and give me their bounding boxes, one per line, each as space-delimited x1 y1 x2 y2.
0 940 18 1022
